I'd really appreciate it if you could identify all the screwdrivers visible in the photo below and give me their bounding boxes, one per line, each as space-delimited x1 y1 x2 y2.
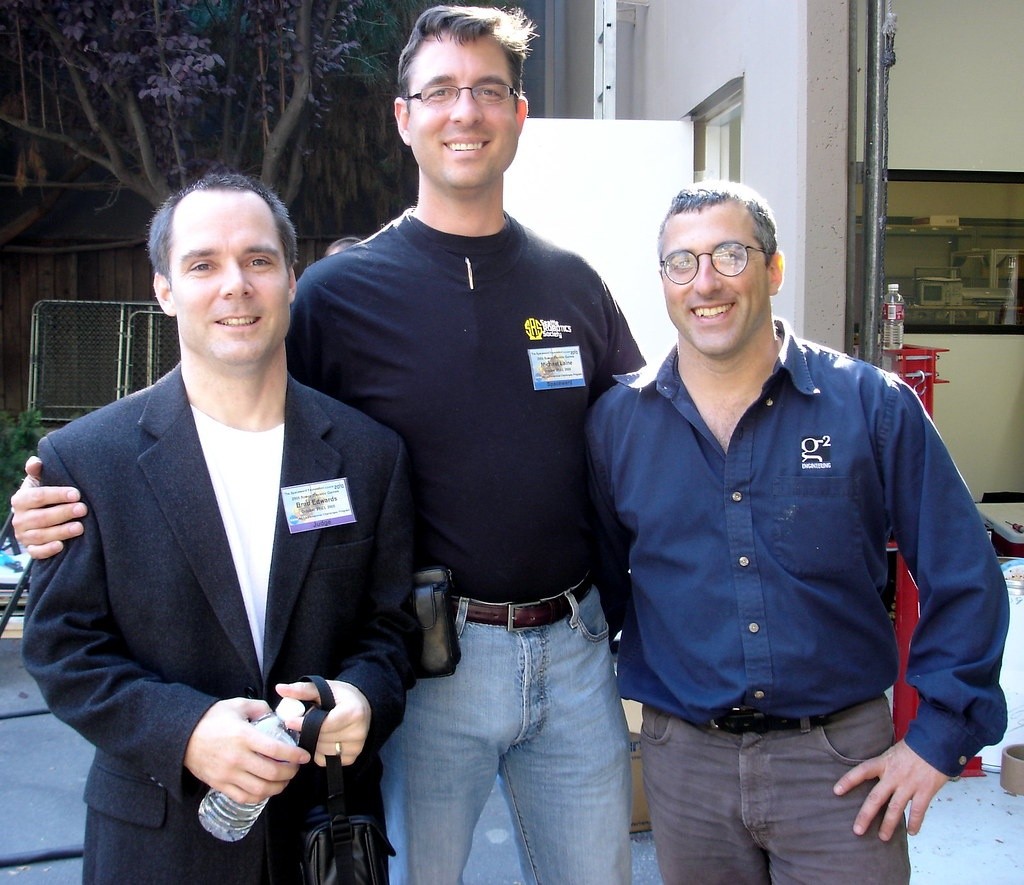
1005 520 1024 534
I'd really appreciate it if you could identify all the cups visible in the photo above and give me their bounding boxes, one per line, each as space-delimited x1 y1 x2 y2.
1000 744 1024 796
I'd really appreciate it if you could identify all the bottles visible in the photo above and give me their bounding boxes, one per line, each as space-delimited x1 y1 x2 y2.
884 283 904 350
197 696 305 842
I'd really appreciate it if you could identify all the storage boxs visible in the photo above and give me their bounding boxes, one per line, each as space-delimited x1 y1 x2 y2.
620 696 650 833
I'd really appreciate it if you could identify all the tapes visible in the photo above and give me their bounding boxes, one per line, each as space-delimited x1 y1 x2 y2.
1000 743 1024 797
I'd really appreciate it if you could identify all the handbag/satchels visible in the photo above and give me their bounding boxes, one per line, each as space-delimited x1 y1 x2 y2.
274 675 396 885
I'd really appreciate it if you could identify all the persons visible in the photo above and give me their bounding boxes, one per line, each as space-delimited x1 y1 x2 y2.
323 237 362 257
20 167 422 884
583 180 1012 885
9 0 655 884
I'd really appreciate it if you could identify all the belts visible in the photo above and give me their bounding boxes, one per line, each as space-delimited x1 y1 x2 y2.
680 705 833 735
452 568 593 628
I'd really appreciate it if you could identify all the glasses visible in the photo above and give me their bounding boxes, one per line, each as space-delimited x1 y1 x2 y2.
660 243 766 285
402 84 519 106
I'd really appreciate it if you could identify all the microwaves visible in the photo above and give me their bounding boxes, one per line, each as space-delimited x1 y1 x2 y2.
917 283 963 306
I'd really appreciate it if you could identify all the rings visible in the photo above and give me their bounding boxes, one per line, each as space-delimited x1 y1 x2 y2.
334 743 342 755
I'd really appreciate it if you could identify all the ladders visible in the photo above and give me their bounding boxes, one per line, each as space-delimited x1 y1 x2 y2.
592 0 618 121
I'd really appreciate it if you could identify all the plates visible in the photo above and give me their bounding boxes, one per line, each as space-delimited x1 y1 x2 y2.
1001 559 1024 585
